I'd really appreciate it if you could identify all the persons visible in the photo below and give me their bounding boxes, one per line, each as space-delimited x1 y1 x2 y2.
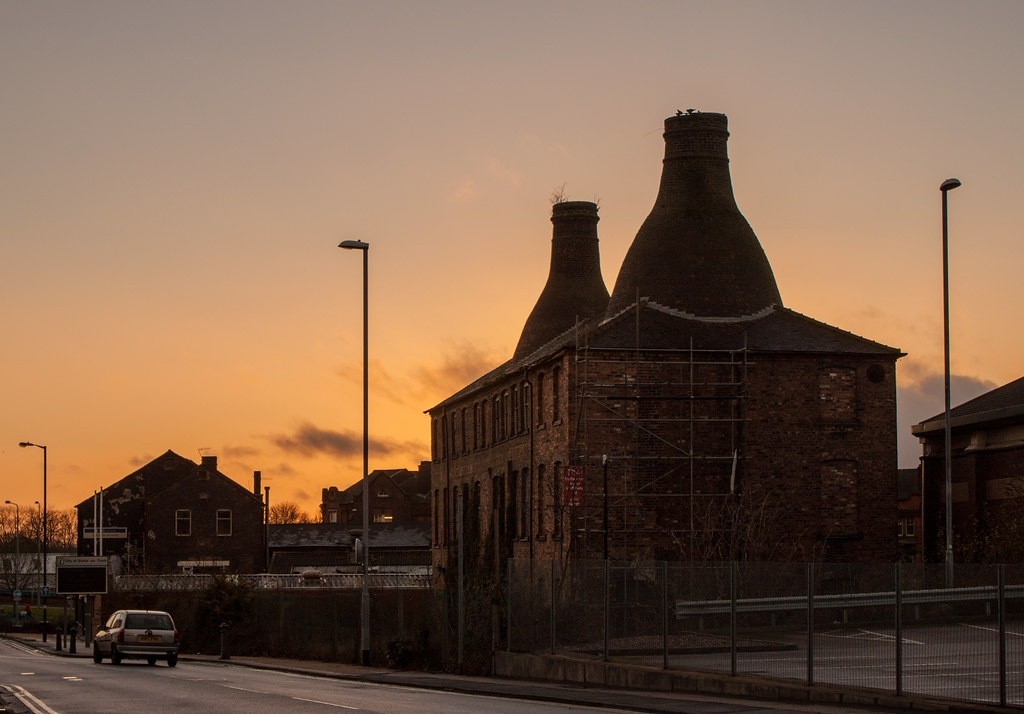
24 605 31 616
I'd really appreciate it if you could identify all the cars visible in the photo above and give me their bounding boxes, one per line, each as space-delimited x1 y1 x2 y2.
92 609 180 668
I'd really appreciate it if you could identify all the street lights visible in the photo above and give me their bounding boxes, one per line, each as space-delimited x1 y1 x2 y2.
940 179 962 590
339 241 371 666
5 500 18 617
35 501 46 605
18 441 47 642
